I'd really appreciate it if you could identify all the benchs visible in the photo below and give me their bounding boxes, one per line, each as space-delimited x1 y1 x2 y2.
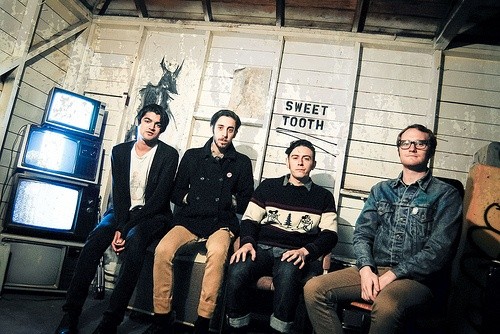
128 243 229 332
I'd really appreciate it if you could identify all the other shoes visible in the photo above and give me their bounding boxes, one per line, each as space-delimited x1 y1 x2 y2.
143 314 172 334
92 321 117 334
192 316 210 334
56 313 79 334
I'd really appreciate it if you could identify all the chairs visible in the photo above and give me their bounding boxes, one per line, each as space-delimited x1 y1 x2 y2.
348 177 463 322
219 251 333 334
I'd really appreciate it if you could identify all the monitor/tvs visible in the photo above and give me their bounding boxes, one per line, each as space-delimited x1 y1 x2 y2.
12 123 104 185
0 233 86 295
41 88 107 141
3 173 100 244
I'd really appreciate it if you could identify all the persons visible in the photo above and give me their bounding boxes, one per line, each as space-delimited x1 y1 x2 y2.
144 110 254 334
53 104 179 334
225 139 338 334
303 125 463 334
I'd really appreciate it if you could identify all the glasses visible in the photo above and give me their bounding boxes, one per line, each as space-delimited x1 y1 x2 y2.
398 140 431 150
